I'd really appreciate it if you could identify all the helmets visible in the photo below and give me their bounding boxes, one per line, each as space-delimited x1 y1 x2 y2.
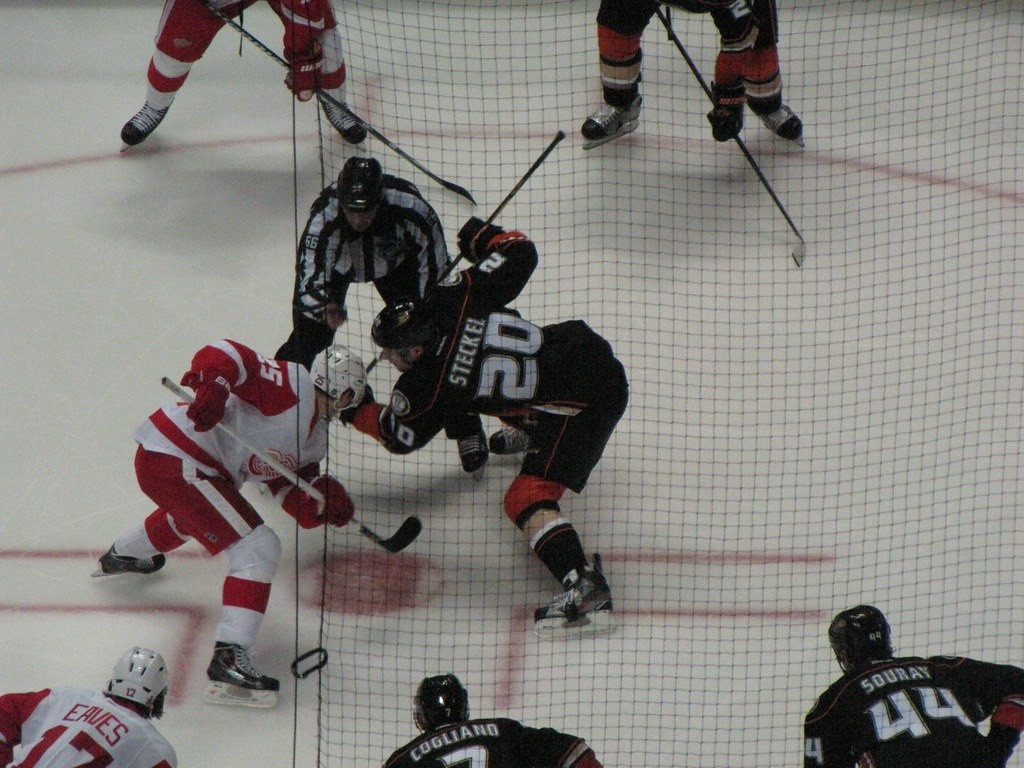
102 645 169 720
370 297 436 348
336 156 382 213
310 344 368 408
826 604 896 674
412 673 471 733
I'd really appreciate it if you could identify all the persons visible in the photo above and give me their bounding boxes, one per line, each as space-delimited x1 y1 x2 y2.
120 0 369 154
90 338 366 709
272 156 489 481
382 674 602 768
581 0 807 150
804 604 1024 768
0 646 176 768
339 215 629 640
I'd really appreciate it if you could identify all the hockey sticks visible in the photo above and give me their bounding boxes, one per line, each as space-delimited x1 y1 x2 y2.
201 0 480 208
161 375 425 554
654 8 809 271
364 130 566 372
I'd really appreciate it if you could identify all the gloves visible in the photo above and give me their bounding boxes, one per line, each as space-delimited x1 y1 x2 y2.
706 76 745 141
284 41 322 102
306 474 355 527
981 719 1021 768
338 384 375 426
456 216 505 263
186 367 233 432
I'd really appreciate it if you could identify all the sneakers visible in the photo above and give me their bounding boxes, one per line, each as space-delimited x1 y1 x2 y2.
202 641 281 709
318 96 368 153
89 542 166 577
118 98 173 152
745 66 806 147
582 71 642 149
534 553 618 638
488 427 536 455
457 429 490 483
